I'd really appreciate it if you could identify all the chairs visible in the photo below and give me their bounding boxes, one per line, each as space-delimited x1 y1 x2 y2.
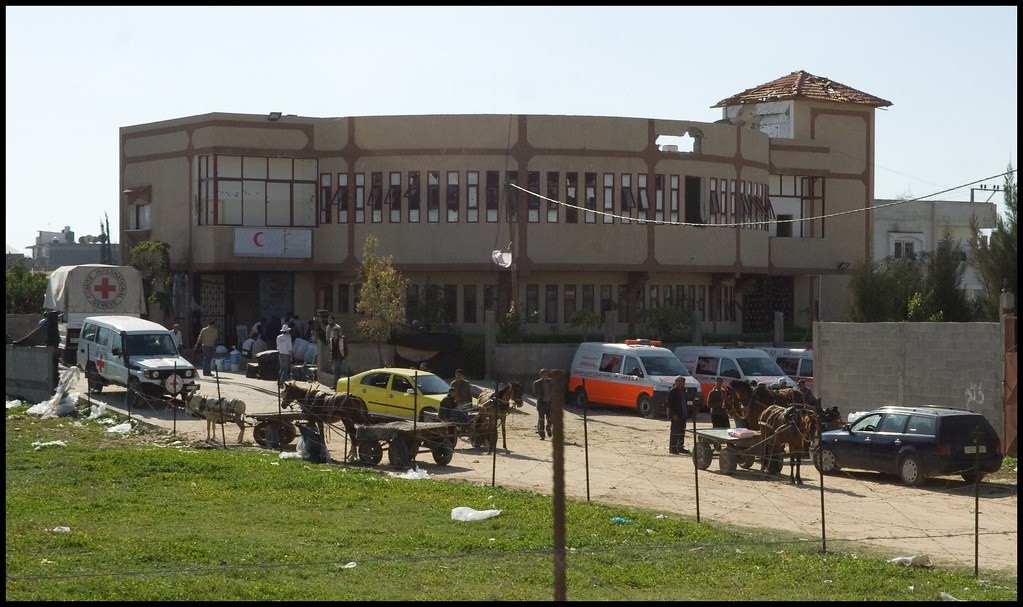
393 379 407 390
883 418 895 431
915 423 931 433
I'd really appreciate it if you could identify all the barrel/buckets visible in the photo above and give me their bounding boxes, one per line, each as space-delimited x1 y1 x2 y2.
211 352 240 372
291 335 319 383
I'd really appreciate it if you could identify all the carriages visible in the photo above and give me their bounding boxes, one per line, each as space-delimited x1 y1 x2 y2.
723 381 822 431
423 381 525 456
685 405 833 488
281 381 457 470
184 391 303 447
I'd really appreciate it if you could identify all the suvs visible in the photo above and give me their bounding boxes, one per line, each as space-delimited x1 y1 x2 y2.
810 405 1003 487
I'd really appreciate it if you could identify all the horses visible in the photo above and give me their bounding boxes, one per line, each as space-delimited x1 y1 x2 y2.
758 404 820 485
479 381 523 455
280 381 369 462
722 379 817 459
184 394 247 444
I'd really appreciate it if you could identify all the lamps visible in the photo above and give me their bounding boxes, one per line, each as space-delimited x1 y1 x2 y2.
837 261 850 270
267 112 282 122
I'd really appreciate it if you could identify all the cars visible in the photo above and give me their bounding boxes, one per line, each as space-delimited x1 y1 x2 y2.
336 367 478 423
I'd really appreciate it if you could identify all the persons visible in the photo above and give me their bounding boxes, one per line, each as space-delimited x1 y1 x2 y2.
169 324 183 358
438 387 458 450
410 363 432 374
276 324 293 389
533 369 552 440
329 328 349 390
450 369 472 406
667 376 691 453
38 311 48 325
242 312 344 360
707 377 818 429
194 320 219 376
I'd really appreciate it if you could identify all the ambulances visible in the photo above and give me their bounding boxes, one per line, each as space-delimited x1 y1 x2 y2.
673 341 796 407
755 347 813 386
76 316 198 408
569 338 702 416
44 265 142 366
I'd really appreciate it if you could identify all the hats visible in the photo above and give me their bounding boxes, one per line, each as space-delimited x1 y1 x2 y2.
778 378 786 386
280 324 291 331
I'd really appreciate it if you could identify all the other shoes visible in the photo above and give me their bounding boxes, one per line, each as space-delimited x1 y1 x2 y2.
669 450 679 454
546 430 552 437
206 374 214 376
538 431 544 439
681 448 691 454
